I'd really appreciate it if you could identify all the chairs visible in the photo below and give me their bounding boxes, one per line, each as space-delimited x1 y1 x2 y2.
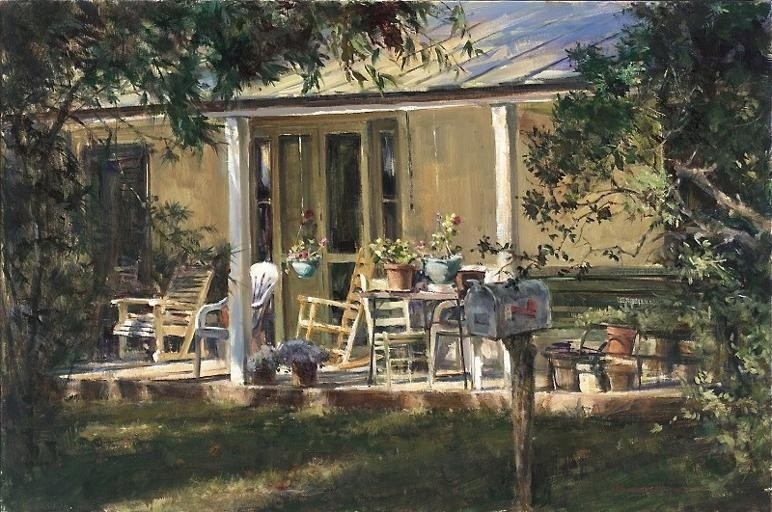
194 261 279 379
358 274 435 393
297 248 382 373
430 299 471 383
110 267 216 363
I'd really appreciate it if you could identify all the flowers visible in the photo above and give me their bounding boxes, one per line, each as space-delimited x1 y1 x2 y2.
426 213 462 257
285 210 327 274
370 237 426 265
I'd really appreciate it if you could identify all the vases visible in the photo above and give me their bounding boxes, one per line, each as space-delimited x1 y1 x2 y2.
542 325 703 393
456 271 484 294
292 260 318 280
425 257 460 284
384 264 414 291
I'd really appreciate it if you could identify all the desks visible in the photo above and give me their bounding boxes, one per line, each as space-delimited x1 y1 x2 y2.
360 290 468 390
